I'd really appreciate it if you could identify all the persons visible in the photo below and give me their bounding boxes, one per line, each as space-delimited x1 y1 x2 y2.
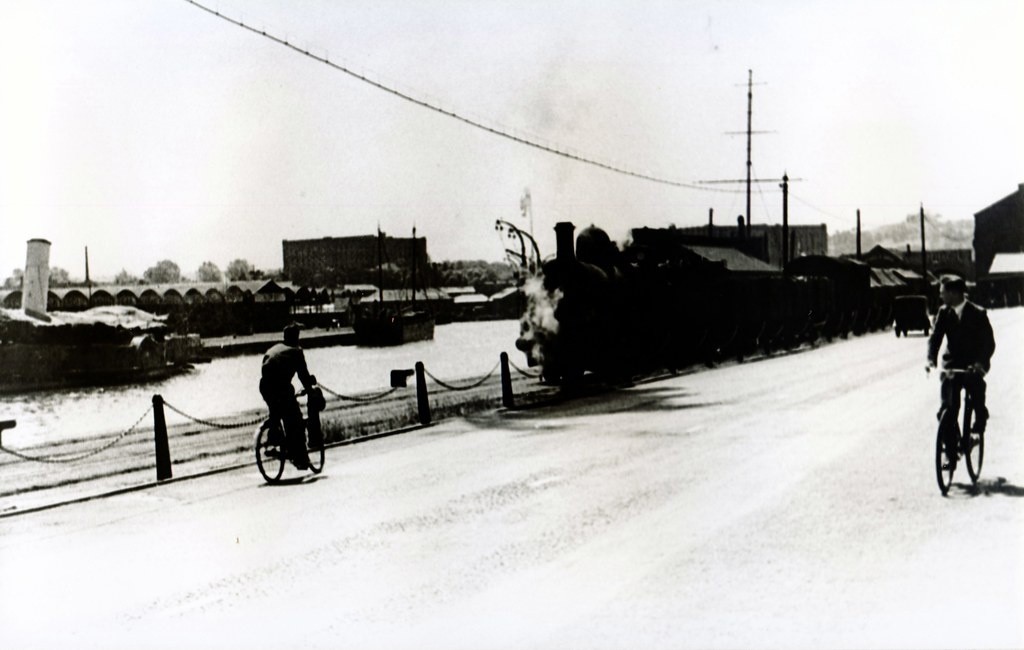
260 325 316 470
926 274 995 462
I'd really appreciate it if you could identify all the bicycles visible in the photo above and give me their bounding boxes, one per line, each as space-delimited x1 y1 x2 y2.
252 385 325 482
926 365 984 492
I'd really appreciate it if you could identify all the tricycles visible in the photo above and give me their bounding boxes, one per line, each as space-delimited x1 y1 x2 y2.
892 295 931 337
